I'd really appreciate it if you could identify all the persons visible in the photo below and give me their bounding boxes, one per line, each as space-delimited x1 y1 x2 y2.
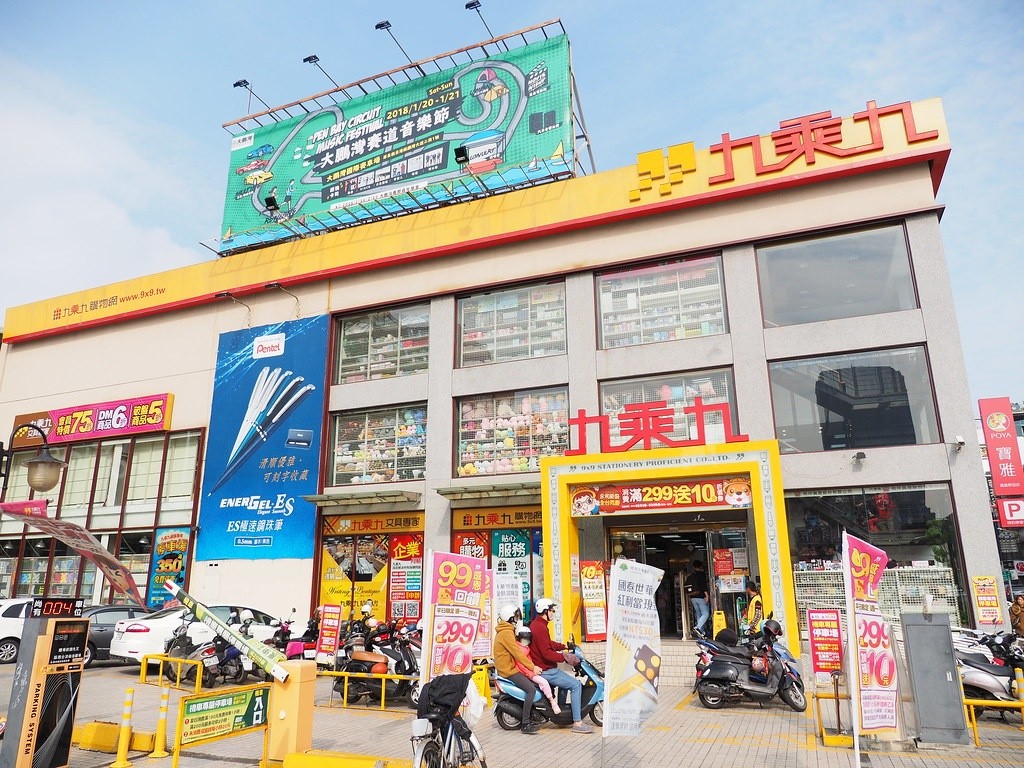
886 559 897 569
745 581 762 636
1009 595 1024 638
685 559 710 639
528 598 595 733
493 603 543 733
827 544 842 561
514 626 561 715
657 582 669 632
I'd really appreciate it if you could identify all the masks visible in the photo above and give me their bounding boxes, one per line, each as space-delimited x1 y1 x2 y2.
520 639 531 646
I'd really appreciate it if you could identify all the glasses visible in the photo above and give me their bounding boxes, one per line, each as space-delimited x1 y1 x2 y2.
547 609 555 613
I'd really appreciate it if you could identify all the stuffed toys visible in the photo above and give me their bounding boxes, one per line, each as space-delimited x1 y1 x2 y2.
333 387 696 484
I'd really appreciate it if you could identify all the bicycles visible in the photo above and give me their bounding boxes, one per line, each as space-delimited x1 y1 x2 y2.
408 670 489 768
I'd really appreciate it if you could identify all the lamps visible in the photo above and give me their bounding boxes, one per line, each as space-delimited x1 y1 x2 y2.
852 452 867 460
303 55 350 100
454 146 487 197
4 541 13 549
266 282 298 302
233 78 283 121
215 291 251 312
465 0 502 54
264 196 305 238
36 540 46 548
375 20 422 77
139 535 150 544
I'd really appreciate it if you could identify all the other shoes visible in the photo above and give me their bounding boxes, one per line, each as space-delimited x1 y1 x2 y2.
550 701 562 715
521 723 539 734
693 628 705 639
573 721 594 733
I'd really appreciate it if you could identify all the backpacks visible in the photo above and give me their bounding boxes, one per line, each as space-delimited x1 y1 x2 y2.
685 572 701 595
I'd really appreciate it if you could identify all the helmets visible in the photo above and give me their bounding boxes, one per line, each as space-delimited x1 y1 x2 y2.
763 620 782 636
751 657 766 672
239 609 254 624
515 625 531 637
361 605 371 616
500 605 518 621
534 598 556 613
416 618 423 631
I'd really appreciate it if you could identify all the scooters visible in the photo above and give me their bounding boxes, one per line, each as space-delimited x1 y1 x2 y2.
953 616 1024 725
691 611 808 712
490 632 604 731
210 598 425 709
162 611 221 688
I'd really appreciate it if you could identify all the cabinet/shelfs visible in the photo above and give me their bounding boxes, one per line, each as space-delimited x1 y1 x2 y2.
459 390 571 476
674 576 694 635
0 554 150 606
460 282 568 367
598 261 726 348
601 368 733 452
332 403 427 487
797 527 843 564
793 566 962 656
338 305 429 385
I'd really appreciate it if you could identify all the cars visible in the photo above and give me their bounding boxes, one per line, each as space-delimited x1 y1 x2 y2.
108 604 294 667
81 606 163 669
0 598 34 664
